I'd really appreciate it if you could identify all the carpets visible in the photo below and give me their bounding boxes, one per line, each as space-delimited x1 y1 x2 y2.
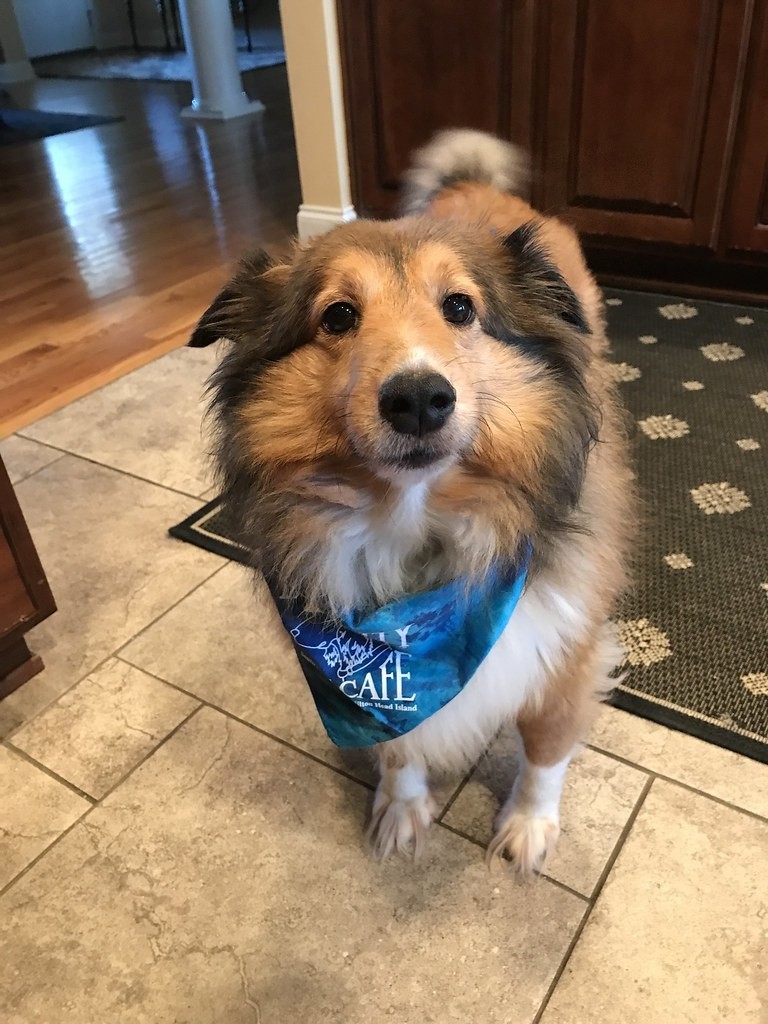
168 288 767 765
0 105 125 145
29 0 286 83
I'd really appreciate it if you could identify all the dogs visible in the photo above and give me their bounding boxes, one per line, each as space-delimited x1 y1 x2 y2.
183 128 636 880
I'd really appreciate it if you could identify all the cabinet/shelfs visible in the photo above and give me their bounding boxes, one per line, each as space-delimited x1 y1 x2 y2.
0 457 57 700
720 0 768 262
335 0 753 249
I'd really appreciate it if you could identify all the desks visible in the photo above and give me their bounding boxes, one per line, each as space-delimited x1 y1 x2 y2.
127 0 251 52
